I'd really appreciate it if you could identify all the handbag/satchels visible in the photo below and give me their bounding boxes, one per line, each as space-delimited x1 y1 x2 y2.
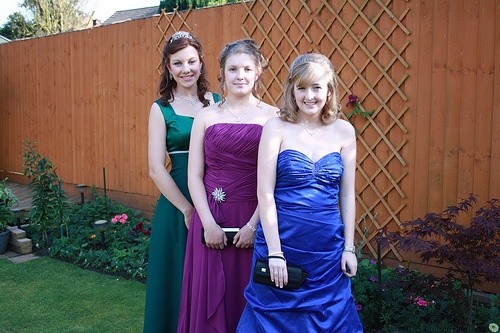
201 227 241 246
255 256 308 289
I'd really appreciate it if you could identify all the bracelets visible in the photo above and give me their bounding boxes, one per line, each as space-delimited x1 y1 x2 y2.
268 251 284 255
247 222 256 231
343 246 356 253
268 256 285 261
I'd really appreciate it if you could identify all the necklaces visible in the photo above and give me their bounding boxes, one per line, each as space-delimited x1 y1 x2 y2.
184 97 199 104
301 121 323 136
226 103 247 122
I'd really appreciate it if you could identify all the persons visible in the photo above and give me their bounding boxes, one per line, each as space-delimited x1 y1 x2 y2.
176 37 280 333
144 31 222 333
235 54 363 333
